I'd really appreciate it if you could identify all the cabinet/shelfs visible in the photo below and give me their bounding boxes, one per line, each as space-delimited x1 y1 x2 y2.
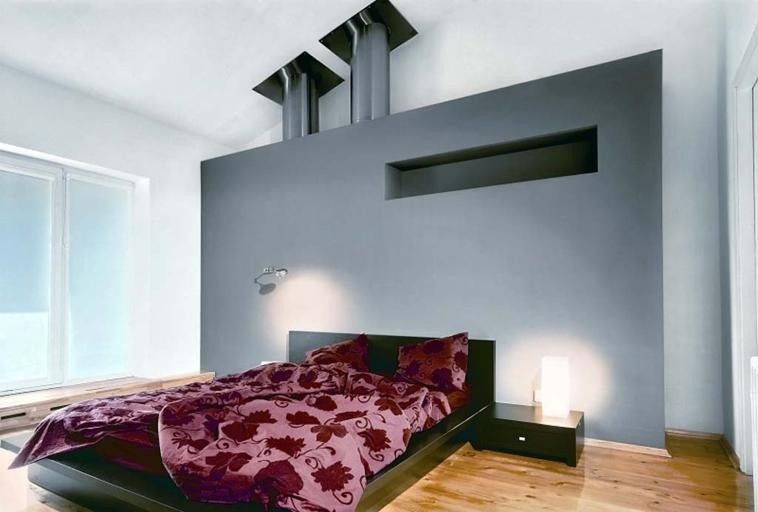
0 372 216 431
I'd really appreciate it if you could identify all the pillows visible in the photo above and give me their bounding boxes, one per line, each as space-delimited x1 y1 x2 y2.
305 333 370 373
392 332 468 392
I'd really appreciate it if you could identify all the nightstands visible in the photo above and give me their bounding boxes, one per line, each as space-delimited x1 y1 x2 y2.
471 402 585 467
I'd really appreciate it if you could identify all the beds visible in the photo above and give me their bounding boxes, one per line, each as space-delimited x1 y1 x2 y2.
0 330 495 512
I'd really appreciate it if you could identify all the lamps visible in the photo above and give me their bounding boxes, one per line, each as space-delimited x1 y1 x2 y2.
254 266 288 283
